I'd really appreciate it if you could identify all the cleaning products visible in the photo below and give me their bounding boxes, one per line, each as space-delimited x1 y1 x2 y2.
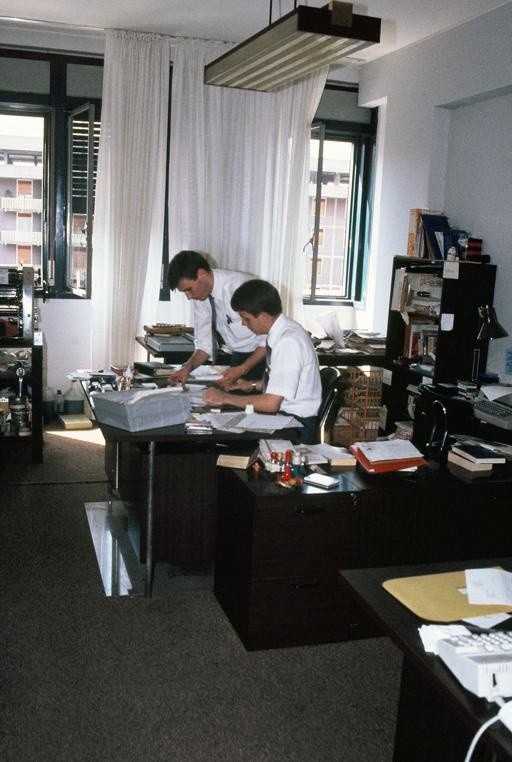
55 389 64 415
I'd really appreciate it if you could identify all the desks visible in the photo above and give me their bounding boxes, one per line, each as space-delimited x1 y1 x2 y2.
336 559 512 761
214 437 511 650
76 337 511 600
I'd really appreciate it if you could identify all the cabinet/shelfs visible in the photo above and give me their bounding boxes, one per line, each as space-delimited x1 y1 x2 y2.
381 259 495 436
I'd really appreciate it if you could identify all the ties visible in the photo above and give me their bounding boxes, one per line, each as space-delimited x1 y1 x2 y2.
208 295 218 365
262 338 272 394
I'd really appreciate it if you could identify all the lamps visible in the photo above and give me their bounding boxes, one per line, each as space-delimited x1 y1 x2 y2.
204 2 384 93
458 302 507 385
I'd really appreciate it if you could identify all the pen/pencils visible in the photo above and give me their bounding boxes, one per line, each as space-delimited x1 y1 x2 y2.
111 376 131 391
226 315 232 324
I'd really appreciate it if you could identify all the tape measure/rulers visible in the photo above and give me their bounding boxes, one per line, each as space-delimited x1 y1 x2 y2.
223 413 246 429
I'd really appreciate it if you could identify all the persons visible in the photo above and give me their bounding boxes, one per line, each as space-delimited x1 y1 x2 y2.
166 251 324 442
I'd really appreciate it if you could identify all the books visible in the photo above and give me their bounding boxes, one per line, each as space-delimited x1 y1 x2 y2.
406 208 469 260
134 361 176 378
447 439 506 472
408 319 439 359
315 328 387 356
216 438 430 475
147 335 195 354
392 267 444 316
